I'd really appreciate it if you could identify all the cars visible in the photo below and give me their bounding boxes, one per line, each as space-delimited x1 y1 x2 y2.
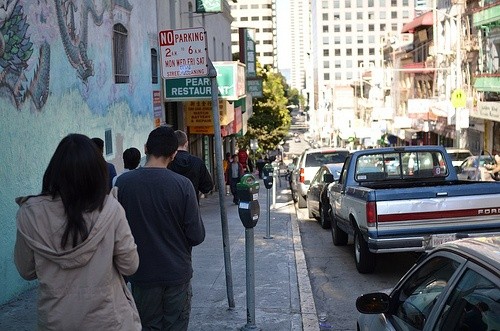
353 232 500 330
282 147 499 231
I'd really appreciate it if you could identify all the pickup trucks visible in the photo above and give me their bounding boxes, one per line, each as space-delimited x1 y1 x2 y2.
326 144 499 275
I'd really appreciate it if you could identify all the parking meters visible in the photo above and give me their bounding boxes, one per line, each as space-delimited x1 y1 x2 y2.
262 163 274 239
235 173 265 331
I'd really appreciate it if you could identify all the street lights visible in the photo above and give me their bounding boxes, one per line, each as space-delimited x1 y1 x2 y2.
414 6 463 147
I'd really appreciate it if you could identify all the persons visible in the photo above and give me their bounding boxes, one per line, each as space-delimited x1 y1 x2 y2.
89 130 214 209
114 126 207 331
256 156 270 179
224 155 243 204
14 134 142 331
484 154 500 176
408 153 416 176
224 152 231 161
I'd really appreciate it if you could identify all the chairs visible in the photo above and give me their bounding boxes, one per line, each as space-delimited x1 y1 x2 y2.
414 167 437 177
331 154 342 163
357 171 389 181
